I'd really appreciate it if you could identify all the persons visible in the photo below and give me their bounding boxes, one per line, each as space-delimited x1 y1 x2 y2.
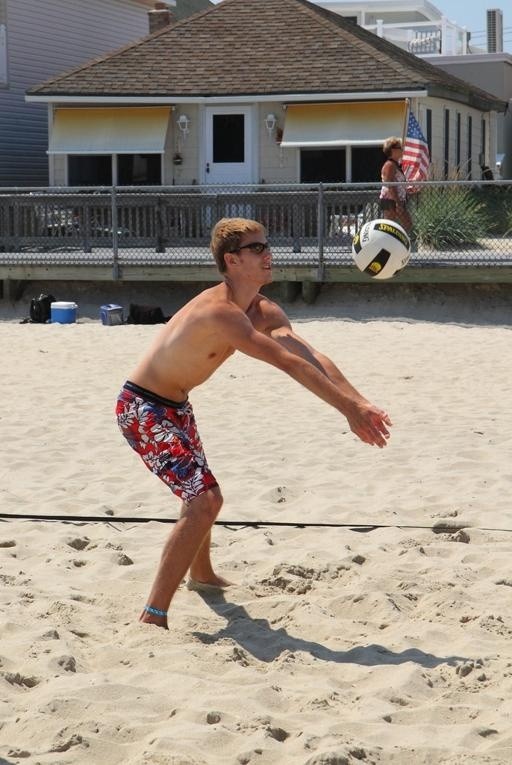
380 137 412 238
113 216 390 630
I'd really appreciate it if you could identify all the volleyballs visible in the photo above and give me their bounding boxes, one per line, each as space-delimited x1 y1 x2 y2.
352 219 410 279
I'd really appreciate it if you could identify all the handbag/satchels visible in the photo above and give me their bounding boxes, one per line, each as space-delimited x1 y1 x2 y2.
128 304 163 323
31 294 56 323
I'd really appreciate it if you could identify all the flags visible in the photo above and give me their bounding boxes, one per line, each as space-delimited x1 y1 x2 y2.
399 108 432 195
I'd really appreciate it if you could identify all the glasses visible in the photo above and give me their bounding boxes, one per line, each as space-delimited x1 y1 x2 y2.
230 242 270 253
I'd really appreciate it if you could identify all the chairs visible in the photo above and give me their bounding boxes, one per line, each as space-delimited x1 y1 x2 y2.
330 201 384 238
20 192 134 253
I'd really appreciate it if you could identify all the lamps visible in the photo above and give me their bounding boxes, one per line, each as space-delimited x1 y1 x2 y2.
263 114 278 139
176 115 191 139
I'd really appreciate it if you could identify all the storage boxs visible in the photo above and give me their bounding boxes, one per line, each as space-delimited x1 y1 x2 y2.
100 304 123 325
50 301 78 324
